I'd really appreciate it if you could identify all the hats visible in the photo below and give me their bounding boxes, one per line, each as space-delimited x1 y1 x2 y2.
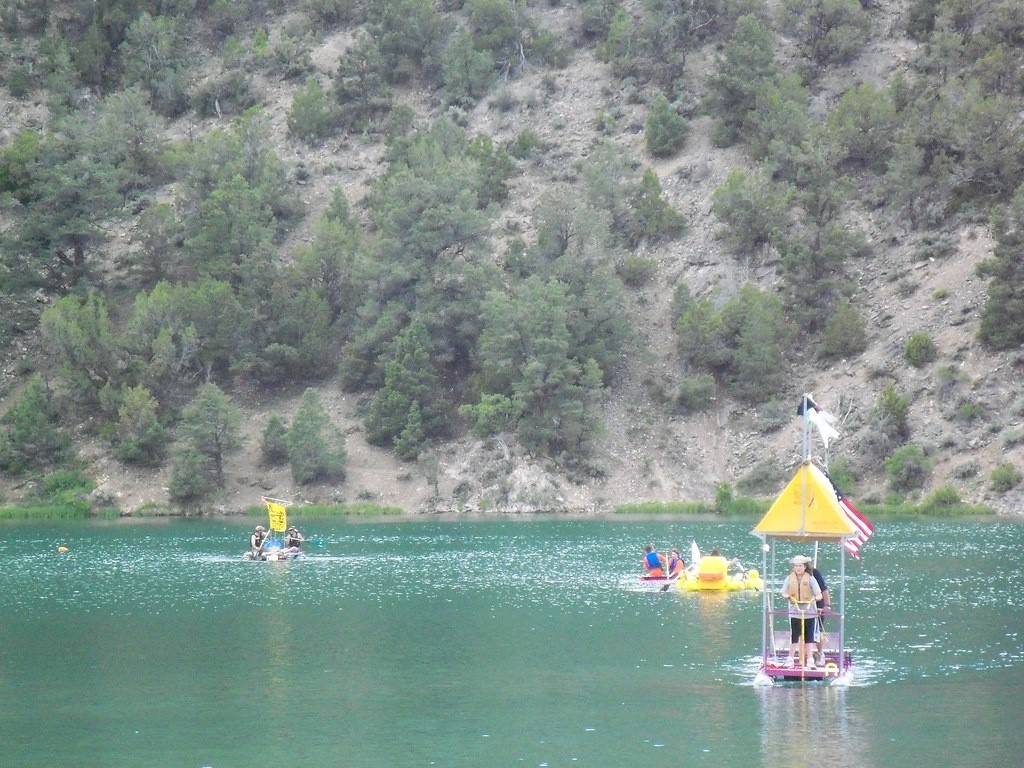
289 527 294 531
256 525 265 531
789 555 812 564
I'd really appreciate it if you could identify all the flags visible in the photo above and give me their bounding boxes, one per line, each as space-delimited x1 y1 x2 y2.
823 467 873 560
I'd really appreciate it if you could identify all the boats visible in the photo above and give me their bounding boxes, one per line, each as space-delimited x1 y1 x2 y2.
756 394 874 681
639 574 682 585
252 496 306 562
677 556 764 592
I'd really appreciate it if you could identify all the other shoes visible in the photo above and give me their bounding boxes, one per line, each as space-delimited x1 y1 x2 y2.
807 658 817 671
783 657 794 669
815 653 825 666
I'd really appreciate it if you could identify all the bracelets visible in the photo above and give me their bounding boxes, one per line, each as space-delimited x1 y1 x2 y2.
826 605 830 607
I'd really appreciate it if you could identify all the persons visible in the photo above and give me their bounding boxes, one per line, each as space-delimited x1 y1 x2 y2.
711 550 737 568
250 525 270 554
282 526 303 554
781 555 831 670
644 546 666 577
668 549 685 580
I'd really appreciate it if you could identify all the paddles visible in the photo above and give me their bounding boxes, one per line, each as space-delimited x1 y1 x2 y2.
732 558 760 591
253 528 270 561
665 551 671 582
659 563 693 593
